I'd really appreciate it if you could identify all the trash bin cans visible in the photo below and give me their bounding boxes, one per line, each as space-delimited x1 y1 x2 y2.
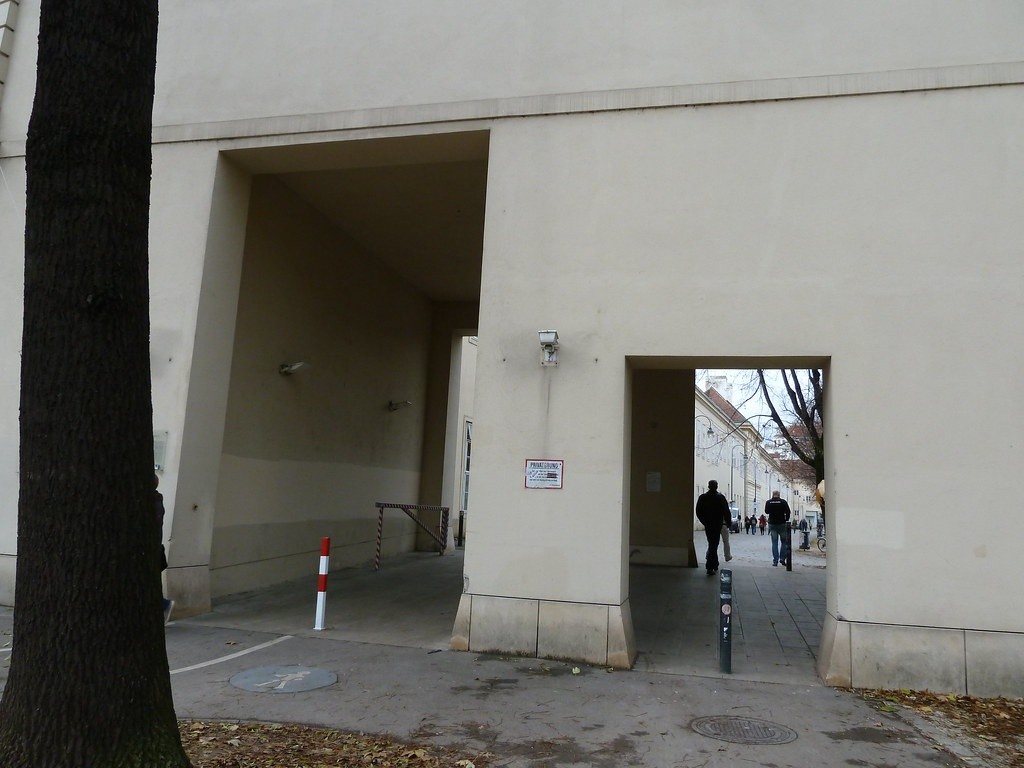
799 532 811 550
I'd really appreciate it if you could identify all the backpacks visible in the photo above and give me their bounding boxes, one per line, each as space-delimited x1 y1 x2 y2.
752 518 756 525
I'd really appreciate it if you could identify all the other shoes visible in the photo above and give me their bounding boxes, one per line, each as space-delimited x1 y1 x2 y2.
714 566 718 571
706 554 708 559
706 569 715 575
772 561 778 566
780 558 786 566
725 556 732 561
164 600 174 625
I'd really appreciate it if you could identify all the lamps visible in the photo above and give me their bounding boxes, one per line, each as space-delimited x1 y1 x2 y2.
539 330 559 353
278 360 304 376
388 400 413 413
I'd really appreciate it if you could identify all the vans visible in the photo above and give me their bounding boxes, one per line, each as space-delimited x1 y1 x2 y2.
728 507 741 533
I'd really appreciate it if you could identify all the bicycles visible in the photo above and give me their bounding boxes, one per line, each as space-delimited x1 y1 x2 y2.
817 533 826 554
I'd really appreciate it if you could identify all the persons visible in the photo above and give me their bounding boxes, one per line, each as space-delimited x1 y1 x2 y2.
744 514 771 535
792 518 812 534
765 490 791 566
696 480 732 576
154 473 176 625
